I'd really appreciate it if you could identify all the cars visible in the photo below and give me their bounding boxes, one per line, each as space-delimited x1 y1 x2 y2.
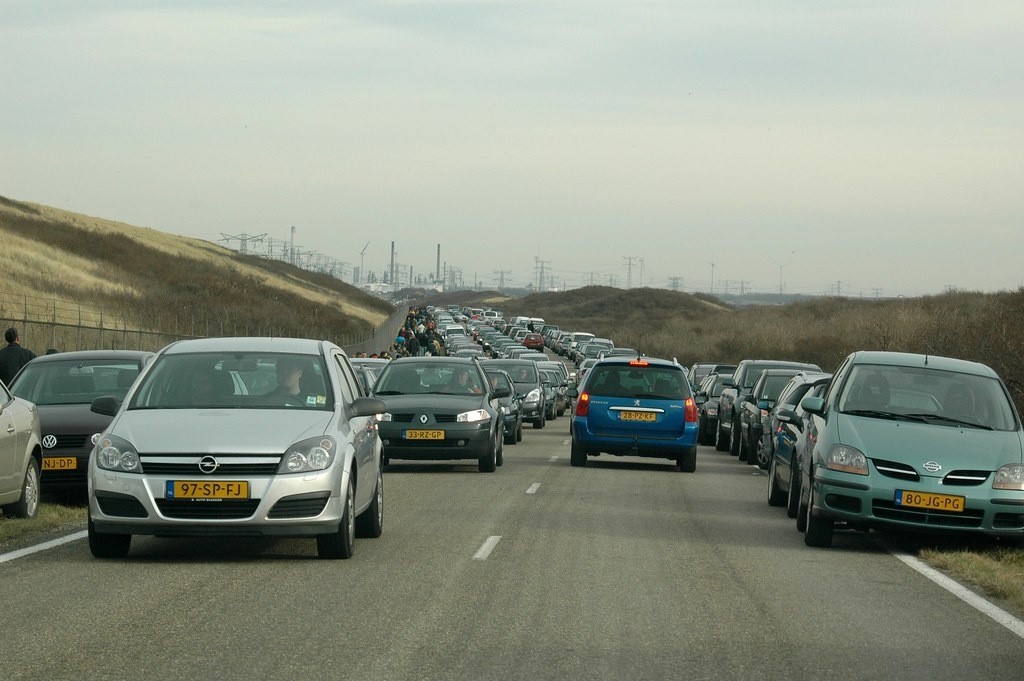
766 377 945 518
85 336 389 559
0 379 44 519
216 303 833 470
564 354 709 472
7 349 158 488
800 348 1024 548
367 355 511 473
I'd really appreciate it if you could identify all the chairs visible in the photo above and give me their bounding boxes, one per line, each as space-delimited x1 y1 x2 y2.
655 379 672 392
860 375 890 405
215 370 234 395
943 384 975 414
50 376 96 394
117 370 139 387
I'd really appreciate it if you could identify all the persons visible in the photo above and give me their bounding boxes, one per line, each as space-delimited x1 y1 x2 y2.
462 309 479 320
188 369 217 405
438 366 480 393
525 321 535 333
351 303 444 360
0 327 37 387
260 357 314 403
489 368 529 388
